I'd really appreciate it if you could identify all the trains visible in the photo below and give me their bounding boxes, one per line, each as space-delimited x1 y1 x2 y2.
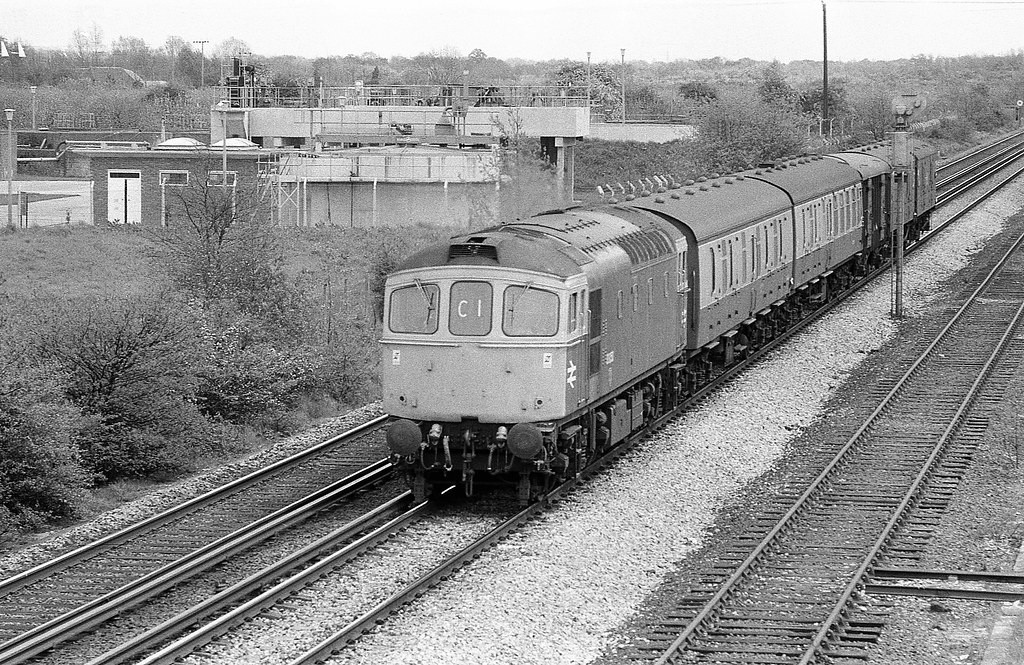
381 131 941 511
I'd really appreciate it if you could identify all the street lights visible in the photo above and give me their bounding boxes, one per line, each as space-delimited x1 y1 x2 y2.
193 41 209 88
